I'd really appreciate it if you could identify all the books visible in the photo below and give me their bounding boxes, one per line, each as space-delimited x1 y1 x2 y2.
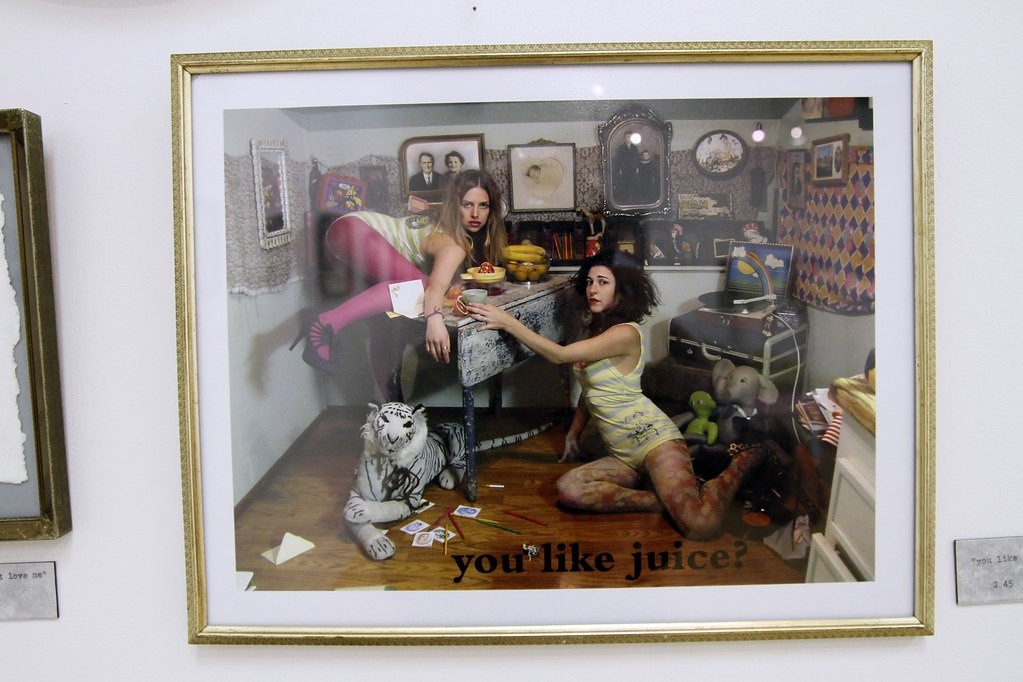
798 389 841 433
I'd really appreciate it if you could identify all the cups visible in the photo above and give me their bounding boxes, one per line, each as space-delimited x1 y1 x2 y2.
462 289 488 309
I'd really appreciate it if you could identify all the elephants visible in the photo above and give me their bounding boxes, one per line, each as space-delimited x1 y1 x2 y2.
711 359 779 444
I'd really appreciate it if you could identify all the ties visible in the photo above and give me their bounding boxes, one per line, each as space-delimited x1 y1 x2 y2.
427 177 431 186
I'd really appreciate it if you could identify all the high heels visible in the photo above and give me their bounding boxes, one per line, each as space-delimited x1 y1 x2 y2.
289 313 338 375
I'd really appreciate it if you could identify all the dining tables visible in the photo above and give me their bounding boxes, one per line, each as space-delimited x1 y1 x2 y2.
385 272 593 504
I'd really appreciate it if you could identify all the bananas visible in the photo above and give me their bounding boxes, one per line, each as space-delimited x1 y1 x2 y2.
501 244 547 263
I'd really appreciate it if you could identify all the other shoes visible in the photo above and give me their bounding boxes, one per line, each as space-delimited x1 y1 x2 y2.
725 438 783 472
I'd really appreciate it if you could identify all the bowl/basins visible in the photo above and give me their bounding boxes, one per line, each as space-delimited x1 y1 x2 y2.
467 266 506 283
502 258 552 286
466 281 506 296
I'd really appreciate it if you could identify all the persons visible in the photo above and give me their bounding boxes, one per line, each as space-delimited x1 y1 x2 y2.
290 170 507 409
444 152 465 185
701 135 737 164
790 161 802 199
409 152 444 190
613 131 659 203
463 249 796 541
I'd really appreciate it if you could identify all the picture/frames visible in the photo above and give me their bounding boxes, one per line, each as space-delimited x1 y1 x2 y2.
0 108 72 541
399 132 485 205
692 130 749 180
801 98 869 124
810 133 849 187
508 138 578 213
596 103 680 223
786 149 809 212
168 38 935 646
248 136 292 250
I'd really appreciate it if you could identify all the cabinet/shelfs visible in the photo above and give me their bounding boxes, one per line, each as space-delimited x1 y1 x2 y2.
801 374 878 584
